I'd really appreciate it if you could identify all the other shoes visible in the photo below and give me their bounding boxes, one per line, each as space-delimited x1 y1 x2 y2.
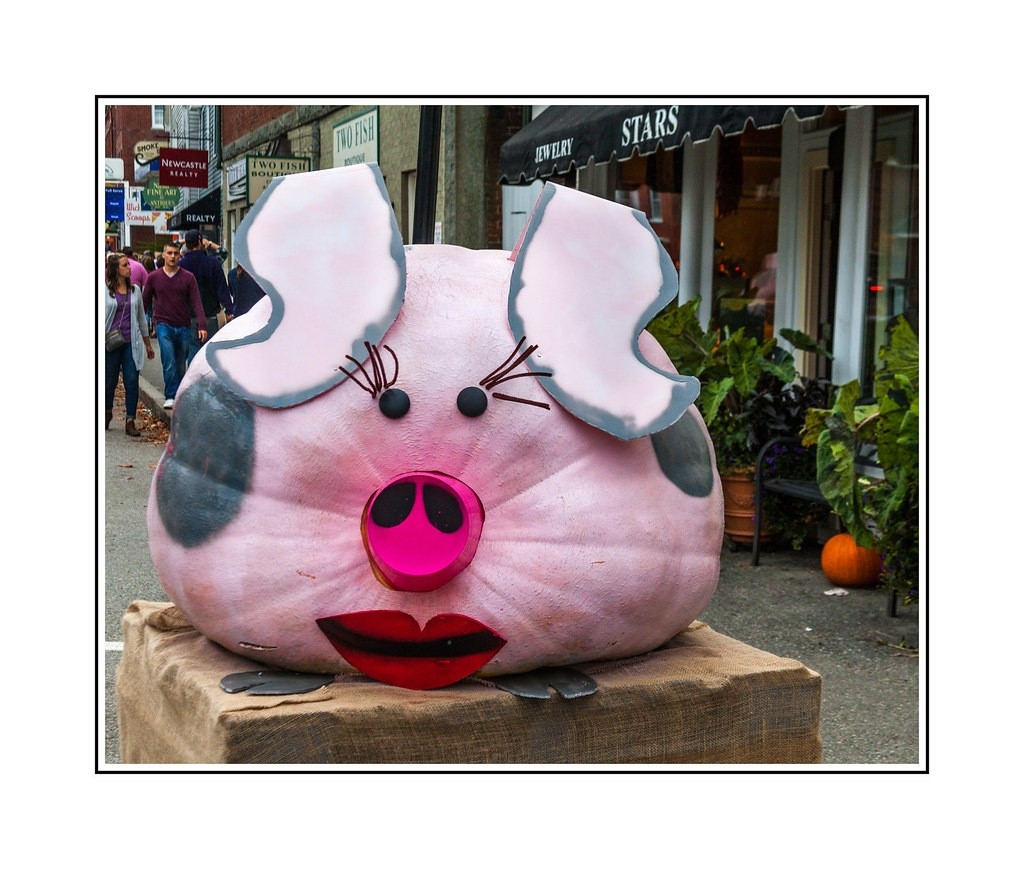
125 420 140 437
163 399 174 409
105 413 112 430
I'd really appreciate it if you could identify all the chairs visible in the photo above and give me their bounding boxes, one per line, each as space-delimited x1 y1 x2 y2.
754 397 898 617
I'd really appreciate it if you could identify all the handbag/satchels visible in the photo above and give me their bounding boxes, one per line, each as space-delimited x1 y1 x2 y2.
105 328 125 352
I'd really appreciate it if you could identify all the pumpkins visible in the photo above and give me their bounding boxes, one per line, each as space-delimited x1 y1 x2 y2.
821 532 883 586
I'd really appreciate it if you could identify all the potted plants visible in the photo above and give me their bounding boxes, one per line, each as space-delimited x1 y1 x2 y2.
646 295 834 554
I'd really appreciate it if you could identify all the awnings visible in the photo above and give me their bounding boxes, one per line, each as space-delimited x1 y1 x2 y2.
497 105 863 186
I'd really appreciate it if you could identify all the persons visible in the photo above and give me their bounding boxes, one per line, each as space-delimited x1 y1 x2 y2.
105 230 266 437
751 253 777 299
142 241 207 409
105 253 153 437
